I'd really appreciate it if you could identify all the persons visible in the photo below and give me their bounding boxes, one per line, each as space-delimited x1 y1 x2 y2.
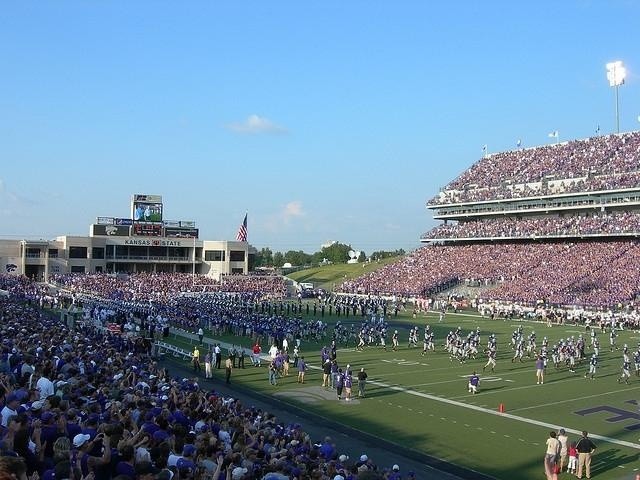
544 428 598 480
417 123 640 396
0 251 418 479
143 205 152 221
136 204 142 219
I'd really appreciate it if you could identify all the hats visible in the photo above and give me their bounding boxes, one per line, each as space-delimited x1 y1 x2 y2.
276 423 322 476
246 448 259 457
7 391 100 448
392 464 399 471
360 455 368 461
339 455 349 462
232 467 248 480
145 408 231 455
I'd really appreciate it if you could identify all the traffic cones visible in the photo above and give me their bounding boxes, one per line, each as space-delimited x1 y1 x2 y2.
500 404 504 413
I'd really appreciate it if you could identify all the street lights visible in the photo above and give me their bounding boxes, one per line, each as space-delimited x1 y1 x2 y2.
605 59 631 135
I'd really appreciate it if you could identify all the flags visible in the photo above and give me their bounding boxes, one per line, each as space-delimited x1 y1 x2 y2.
235 214 247 243
